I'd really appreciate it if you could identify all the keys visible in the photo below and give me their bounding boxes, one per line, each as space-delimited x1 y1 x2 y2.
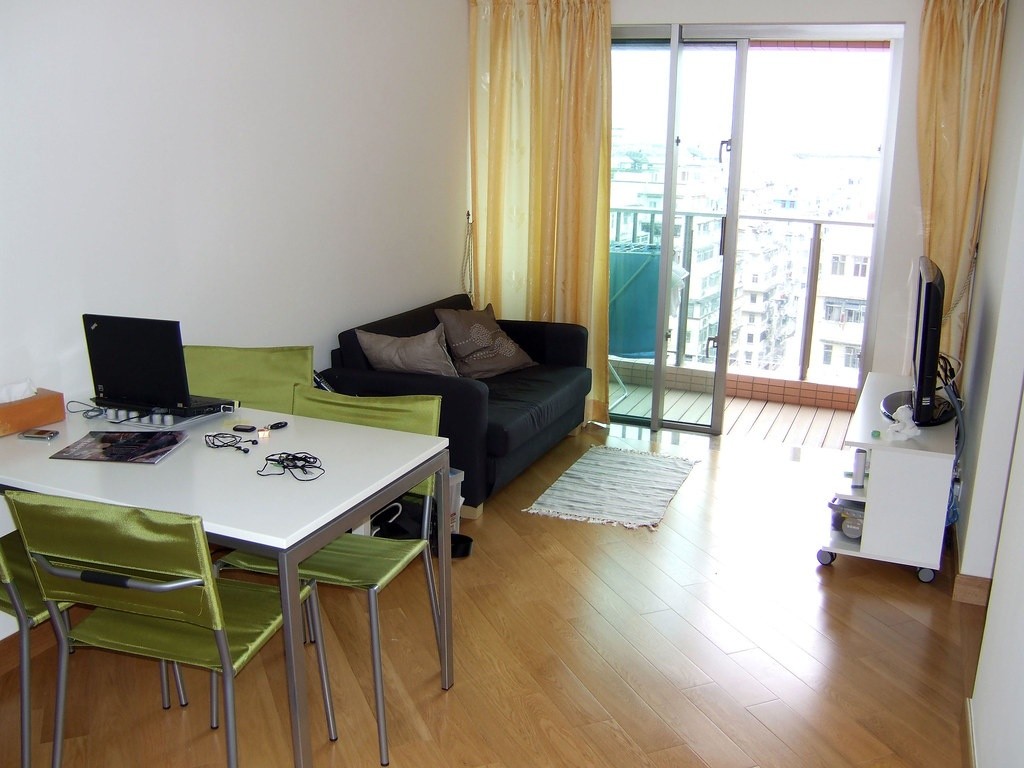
270 421 288 429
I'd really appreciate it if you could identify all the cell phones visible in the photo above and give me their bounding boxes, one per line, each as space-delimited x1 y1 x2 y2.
18 428 59 441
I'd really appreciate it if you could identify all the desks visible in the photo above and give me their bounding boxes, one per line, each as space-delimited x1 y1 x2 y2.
0 391 454 768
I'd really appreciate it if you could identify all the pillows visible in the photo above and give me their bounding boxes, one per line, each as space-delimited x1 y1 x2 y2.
354 323 459 378
434 303 539 380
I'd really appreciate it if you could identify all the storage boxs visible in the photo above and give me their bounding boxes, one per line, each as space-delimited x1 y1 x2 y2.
449 466 464 534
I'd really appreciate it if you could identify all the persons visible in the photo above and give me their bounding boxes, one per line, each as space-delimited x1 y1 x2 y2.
95 434 178 461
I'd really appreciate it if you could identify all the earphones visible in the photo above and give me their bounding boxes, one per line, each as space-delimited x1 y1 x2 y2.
235 446 249 453
306 457 317 464
244 440 258 445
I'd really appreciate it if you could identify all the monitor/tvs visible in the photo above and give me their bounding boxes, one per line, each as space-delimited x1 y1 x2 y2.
879 254 957 427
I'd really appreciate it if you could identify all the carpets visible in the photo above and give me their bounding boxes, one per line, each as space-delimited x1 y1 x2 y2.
521 443 701 532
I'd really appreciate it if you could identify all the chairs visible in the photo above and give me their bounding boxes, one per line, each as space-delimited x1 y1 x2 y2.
1 343 440 768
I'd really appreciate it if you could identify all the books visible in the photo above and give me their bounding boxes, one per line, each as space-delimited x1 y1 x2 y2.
49 430 191 465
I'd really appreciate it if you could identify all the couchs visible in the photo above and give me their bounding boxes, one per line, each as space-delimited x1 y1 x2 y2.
314 293 592 518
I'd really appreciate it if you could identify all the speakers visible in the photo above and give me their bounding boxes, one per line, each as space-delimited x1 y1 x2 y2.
850 448 867 490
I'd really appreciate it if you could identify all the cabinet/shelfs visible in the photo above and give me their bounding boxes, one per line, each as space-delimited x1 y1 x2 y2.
817 370 955 584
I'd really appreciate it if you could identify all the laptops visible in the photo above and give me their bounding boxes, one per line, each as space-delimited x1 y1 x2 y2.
82 313 241 418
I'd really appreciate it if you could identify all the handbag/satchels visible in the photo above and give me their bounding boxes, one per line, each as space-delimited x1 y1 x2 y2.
372 493 473 558
